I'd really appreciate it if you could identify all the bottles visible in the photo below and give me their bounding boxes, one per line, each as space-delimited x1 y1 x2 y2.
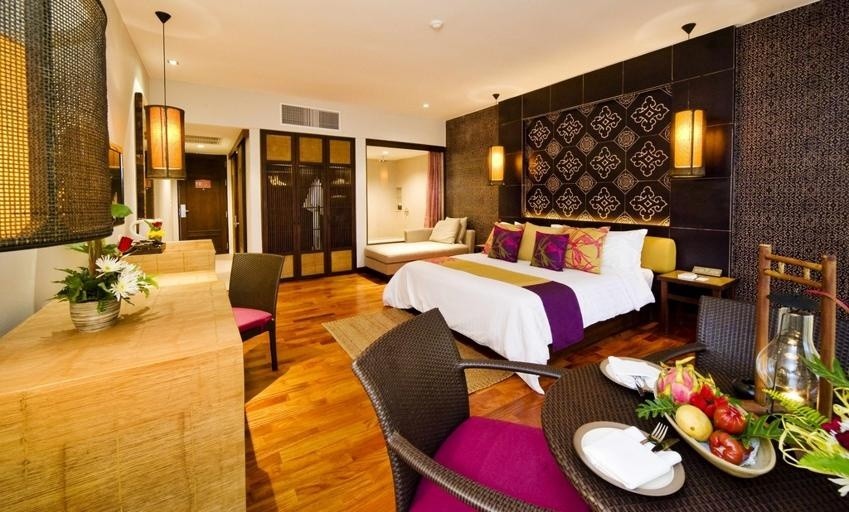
753 307 820 404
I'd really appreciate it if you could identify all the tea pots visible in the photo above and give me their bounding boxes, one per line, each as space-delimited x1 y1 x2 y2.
130 219 158 239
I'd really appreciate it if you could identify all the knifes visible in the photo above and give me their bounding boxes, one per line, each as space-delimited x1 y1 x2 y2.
630 375 644 397
652 437 681 452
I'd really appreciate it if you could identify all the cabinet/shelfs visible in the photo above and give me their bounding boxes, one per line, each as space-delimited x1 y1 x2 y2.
326 135 355 276
261 128 326 280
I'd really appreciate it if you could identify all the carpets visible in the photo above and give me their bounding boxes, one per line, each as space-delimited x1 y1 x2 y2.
319 309 515 398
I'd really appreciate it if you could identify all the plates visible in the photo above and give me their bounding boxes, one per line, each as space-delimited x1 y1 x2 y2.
572 420 687 496
654 374 777 478
598 356 664 394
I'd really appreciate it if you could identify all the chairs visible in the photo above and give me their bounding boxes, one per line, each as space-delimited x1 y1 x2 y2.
348 307 597 512
229 251 285 371
695 293 849 420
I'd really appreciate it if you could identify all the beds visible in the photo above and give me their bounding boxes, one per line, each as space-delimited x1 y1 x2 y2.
380 216 679 397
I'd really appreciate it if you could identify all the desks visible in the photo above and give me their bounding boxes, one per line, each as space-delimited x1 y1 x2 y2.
0 238 251 512
654 270 739 336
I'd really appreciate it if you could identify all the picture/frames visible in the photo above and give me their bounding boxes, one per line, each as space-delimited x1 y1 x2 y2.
108 142 125 226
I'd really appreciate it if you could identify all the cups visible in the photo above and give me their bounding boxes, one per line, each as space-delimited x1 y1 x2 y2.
117 237 133 251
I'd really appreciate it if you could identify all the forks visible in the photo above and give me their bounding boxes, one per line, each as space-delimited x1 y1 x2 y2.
639 421 669 445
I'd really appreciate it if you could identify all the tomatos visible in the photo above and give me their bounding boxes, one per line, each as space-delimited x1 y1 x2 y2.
709 430 751 466
714 404 746 435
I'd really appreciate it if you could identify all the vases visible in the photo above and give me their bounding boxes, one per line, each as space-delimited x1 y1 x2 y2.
69 297 120 334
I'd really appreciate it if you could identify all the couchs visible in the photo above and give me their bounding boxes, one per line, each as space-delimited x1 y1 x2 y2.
363 227 476 277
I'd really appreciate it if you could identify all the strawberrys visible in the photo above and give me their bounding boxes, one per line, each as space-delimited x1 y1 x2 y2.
690 386 728 415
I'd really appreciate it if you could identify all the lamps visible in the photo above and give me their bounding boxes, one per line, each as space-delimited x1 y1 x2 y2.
488 94 506 187
146 10 187 181
668 22 706 180
754 292 823 428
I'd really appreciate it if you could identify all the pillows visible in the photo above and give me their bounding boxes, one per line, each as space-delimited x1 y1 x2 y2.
481 220 649 277
444 216 469 244
428 219 460 244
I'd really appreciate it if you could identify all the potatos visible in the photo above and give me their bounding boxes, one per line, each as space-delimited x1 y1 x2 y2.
673 404 713 442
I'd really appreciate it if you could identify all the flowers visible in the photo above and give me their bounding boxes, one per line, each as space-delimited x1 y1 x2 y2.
45 203 160 315
760 353 849 498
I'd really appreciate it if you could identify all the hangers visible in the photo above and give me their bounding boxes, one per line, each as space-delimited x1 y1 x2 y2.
267 171 287 187
